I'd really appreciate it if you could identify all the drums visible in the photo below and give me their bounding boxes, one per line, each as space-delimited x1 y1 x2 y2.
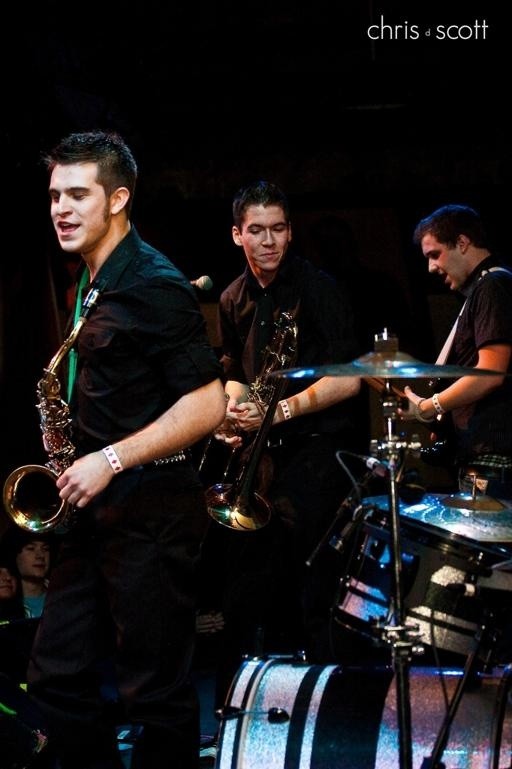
218 651 346 768
308 501 511 664
373 660 512 769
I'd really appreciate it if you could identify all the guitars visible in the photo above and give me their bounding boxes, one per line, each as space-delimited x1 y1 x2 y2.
366 377 455 440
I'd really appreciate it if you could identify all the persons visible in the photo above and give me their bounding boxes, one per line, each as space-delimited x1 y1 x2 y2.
1 560 44 768
379 204 510 504
220 180 364 653
194 608 224 635
4 526 101 764
43 131 226 767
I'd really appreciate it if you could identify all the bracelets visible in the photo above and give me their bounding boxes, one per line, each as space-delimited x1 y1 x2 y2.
413 397 434 424
280 400 293 421
432 393 447 417
103 445 123 475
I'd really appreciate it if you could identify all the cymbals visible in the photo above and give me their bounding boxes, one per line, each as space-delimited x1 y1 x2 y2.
268 329 511 378
359 461 511 542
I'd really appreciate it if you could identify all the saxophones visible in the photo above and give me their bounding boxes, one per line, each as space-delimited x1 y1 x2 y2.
199 298 302 530
4 282 105 534
